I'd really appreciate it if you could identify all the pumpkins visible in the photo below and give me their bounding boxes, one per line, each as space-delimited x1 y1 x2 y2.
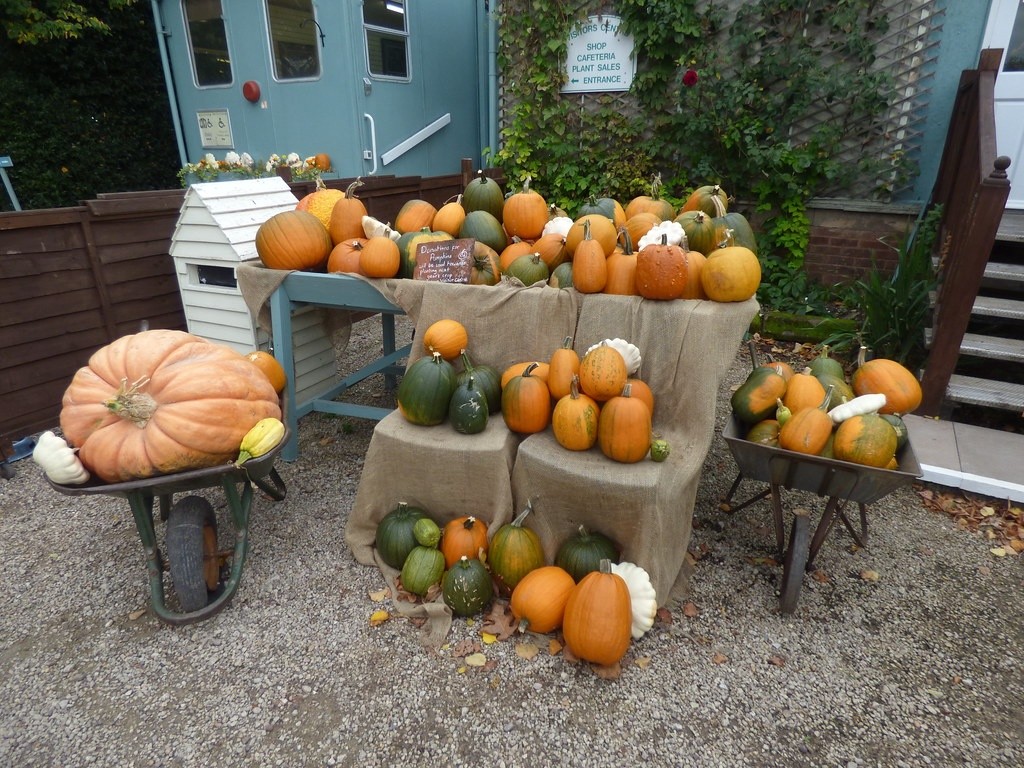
32 329 285 486
397 319 506 434
311 153 330 171
730 340 923 472
500 335 671 462
255 169 760 302
374 496 657 666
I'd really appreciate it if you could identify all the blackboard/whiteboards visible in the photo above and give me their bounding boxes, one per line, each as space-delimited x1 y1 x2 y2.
412 237 475 284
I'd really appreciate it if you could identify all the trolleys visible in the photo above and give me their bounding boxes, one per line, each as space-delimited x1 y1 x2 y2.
721 334 925 614
42 384 295 627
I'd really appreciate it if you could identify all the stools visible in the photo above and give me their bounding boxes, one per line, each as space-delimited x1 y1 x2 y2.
373 409 662 557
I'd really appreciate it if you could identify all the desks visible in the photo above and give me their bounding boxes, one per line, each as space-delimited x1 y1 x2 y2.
236 262 754 462
